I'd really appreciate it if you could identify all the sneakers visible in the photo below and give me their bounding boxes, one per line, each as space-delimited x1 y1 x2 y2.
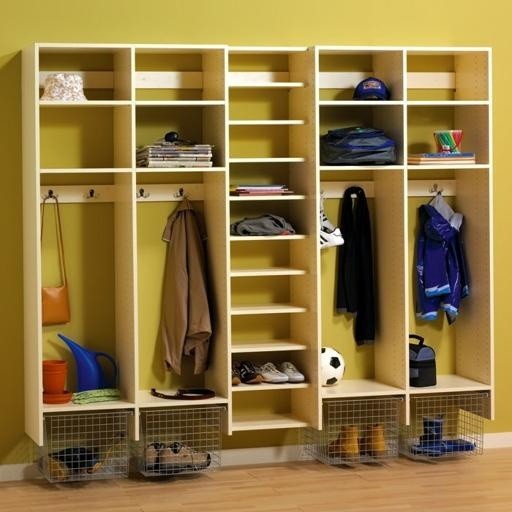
255 362 288 382
159 442 211 469
238 361 263 383
276 361 304 382
232 360 240 384
145 443 165 469
321 208 345 249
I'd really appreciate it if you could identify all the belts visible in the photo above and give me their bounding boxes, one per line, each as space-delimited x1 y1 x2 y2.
151 388 215 399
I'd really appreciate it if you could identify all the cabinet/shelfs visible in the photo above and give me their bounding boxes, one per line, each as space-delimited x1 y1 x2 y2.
21 42 495 447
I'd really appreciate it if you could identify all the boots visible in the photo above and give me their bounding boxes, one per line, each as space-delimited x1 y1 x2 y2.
330 427 359 461
360 424 385 455
412 418 442 455
420 436 474 451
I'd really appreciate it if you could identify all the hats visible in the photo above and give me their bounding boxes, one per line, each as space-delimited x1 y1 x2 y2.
353 77 390 100
40 73 88 102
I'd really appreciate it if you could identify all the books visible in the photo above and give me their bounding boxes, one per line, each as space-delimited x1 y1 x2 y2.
408 152 476 165
234 182 294 196
137 139 213 167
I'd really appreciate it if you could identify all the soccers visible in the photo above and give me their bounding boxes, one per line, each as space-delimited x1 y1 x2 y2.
319 347 345 387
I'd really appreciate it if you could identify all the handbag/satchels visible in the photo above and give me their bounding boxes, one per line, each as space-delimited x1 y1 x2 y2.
320 127 396 165
43 286 70 325
409 334 436 387
